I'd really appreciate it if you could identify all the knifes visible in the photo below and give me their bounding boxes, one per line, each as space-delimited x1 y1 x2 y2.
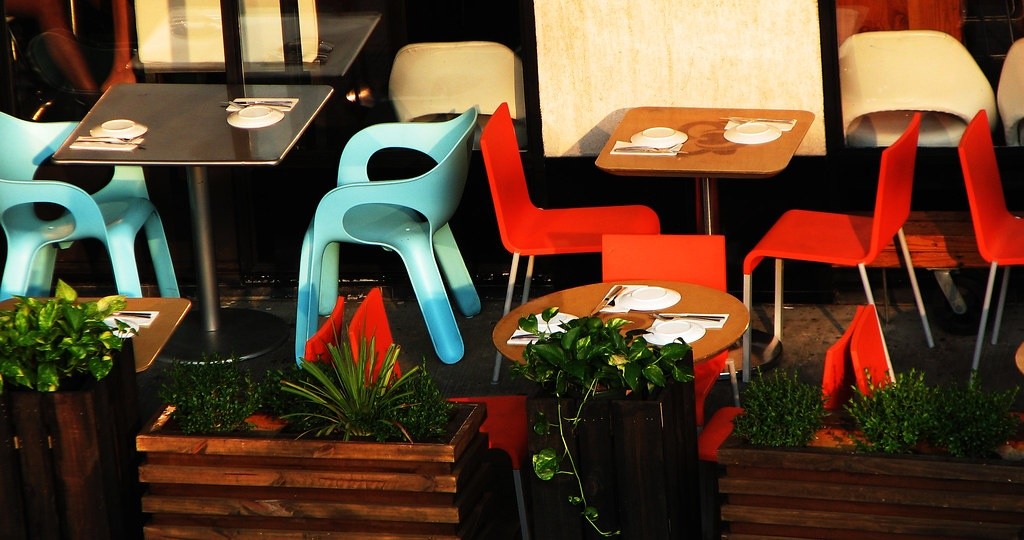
591 285 622 316
720 118 794 122
73 140 142 145
614 149 689 154
219 100 293 104
650 313 725 319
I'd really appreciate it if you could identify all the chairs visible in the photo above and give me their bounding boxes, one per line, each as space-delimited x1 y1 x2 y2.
0 111 180 300
744 111 934 386
480 102 657 310
958 110 1024 386
347 289 533 540
696 303 864 461
852 306 894 405
244 294 345 430
295 107 478 365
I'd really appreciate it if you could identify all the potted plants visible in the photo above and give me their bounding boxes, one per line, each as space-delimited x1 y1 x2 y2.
0 297 140 540
717 365 1024 540
511 308 703 540
134 336 486 540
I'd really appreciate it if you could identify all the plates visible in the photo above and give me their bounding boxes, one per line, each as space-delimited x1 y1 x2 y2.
619 287 682 311
643 320 706 346
226 106 284 129
104 317 140 338
723 123 782 145
518 312 579 334
630 127 688 148
90 119 149 139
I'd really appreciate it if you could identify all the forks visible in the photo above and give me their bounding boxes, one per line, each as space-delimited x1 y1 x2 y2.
624 144 678 150
729 117 793 125
602 287 625 310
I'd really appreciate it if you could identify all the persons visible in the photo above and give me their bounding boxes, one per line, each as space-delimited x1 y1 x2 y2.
5 0 96 91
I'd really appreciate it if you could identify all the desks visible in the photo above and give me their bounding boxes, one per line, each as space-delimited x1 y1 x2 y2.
494 281 750 375
596 108 814 234
51 82 336 367
